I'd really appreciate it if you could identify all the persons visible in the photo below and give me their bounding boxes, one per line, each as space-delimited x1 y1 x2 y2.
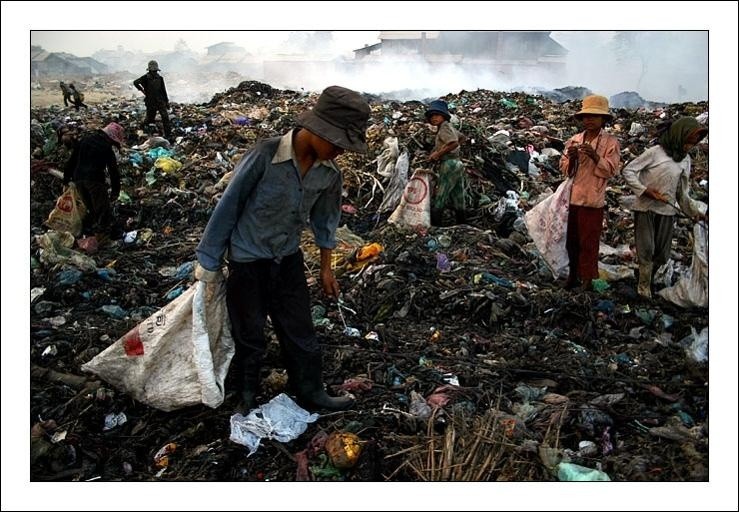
131 59 173 141
68 83 88 111
559 94 621 295
63 121 125 229
423 99 467 218
191 83 374 417
621 116 708 297
58 79 75 107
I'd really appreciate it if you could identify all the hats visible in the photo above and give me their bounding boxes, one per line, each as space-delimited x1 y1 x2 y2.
97 122 126 149
146 61 162 71
574 96 613 123
295 86 370 154
425 101 451 118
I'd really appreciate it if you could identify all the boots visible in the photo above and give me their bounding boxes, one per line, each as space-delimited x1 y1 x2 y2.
297 365 357 411
